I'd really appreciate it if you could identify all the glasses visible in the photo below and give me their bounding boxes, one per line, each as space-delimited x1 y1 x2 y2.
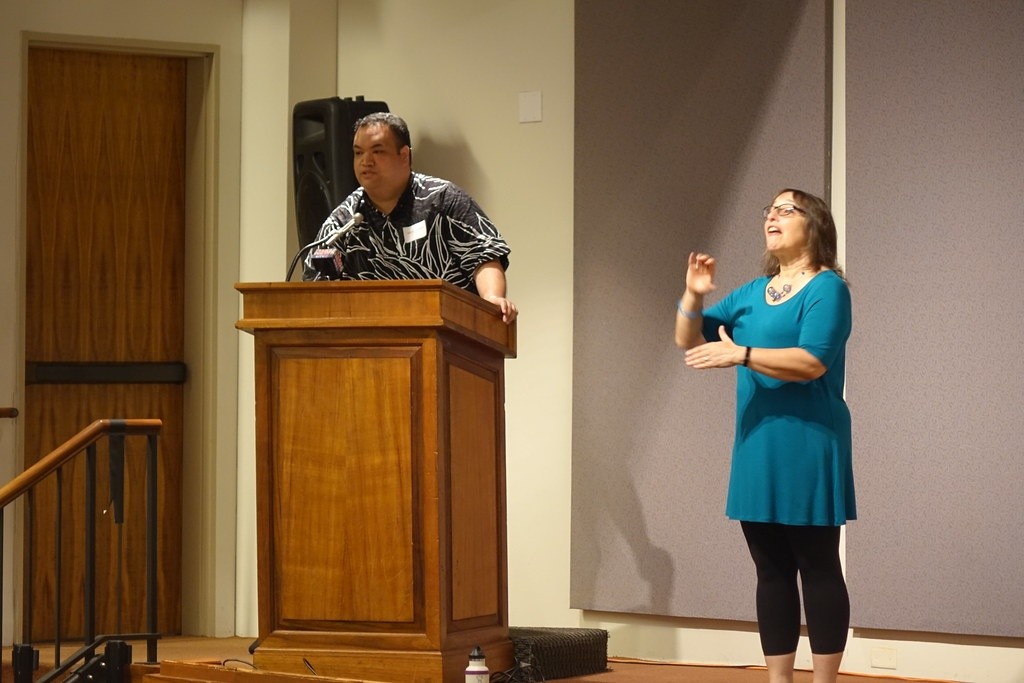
763 204 805 218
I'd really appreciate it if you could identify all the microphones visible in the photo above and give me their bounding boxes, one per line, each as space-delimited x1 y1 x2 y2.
285 212 363 282
312 249 344 282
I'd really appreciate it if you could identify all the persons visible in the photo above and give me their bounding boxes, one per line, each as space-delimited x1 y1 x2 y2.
676 188 857 683
302 113 519 325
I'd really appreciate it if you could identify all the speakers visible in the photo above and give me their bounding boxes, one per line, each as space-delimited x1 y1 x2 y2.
292 96 390 281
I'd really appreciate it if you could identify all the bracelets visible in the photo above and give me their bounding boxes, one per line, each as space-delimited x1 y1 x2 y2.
678 303 700 319
743 346 751 366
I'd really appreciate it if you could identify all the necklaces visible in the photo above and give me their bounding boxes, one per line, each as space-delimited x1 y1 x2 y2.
768 272 805 301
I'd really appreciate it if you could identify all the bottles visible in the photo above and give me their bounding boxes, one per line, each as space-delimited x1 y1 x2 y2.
465 645 489 683
